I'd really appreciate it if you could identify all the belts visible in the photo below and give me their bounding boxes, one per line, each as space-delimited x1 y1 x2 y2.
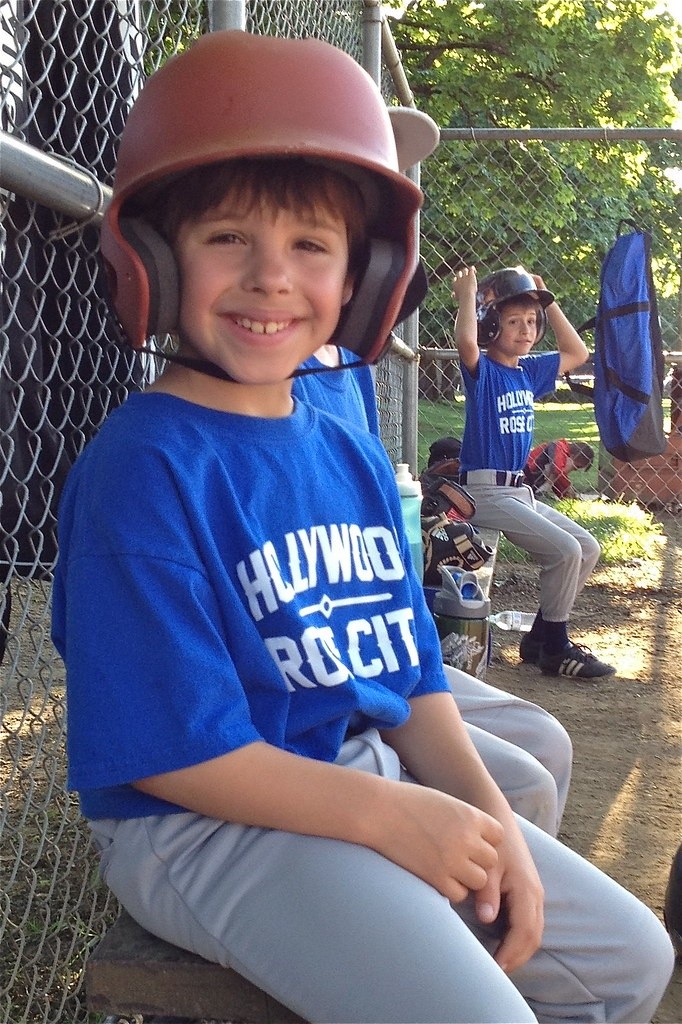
340 716 373 742
457 471 524 488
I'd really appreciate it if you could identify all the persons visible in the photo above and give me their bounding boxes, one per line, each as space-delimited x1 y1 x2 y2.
44 29 682 1024
523 440 595 500
450 265 617 679
291 340 576 842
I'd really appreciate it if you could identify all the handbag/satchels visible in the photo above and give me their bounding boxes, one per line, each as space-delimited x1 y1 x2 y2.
563 218 665 461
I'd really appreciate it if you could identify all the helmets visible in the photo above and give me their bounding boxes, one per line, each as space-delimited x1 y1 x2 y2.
475 268 554 345
96 30 428 364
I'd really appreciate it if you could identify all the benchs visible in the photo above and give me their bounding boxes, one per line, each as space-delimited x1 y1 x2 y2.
422 525 500 681
83 907 309 1024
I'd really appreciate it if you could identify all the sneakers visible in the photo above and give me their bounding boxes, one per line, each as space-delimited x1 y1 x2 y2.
540 639 617 680
518 632 541 663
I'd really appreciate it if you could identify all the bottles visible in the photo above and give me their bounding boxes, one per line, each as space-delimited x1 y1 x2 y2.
394 463 413 483
397 480 424 583
490 611 537 632
